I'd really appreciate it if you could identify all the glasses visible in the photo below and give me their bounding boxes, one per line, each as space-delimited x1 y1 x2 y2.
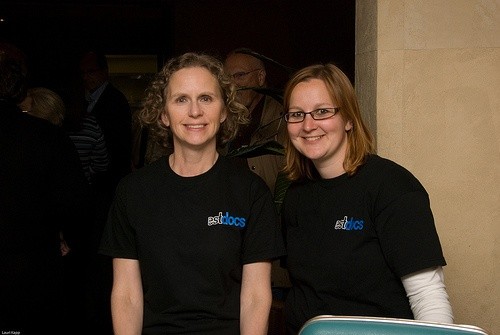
283 106 342 123
224 67 261 79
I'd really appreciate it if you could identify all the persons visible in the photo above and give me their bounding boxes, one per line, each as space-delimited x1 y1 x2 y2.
216 46 305 302
0 47 133 335
98 53 285 335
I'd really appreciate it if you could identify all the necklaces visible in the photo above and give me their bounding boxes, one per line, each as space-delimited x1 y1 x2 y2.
279 64 457 335
